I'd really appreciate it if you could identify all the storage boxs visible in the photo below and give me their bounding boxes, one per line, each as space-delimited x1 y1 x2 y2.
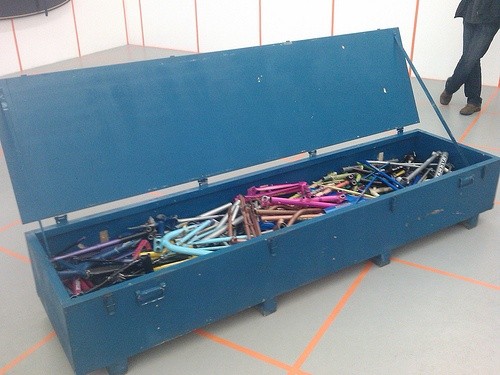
0 27 499 375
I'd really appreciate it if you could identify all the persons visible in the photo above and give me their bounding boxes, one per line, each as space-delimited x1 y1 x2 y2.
439 0 500 115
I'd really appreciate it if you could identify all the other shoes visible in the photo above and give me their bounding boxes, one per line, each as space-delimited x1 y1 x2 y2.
460 103 481 115
440 89 452 105
